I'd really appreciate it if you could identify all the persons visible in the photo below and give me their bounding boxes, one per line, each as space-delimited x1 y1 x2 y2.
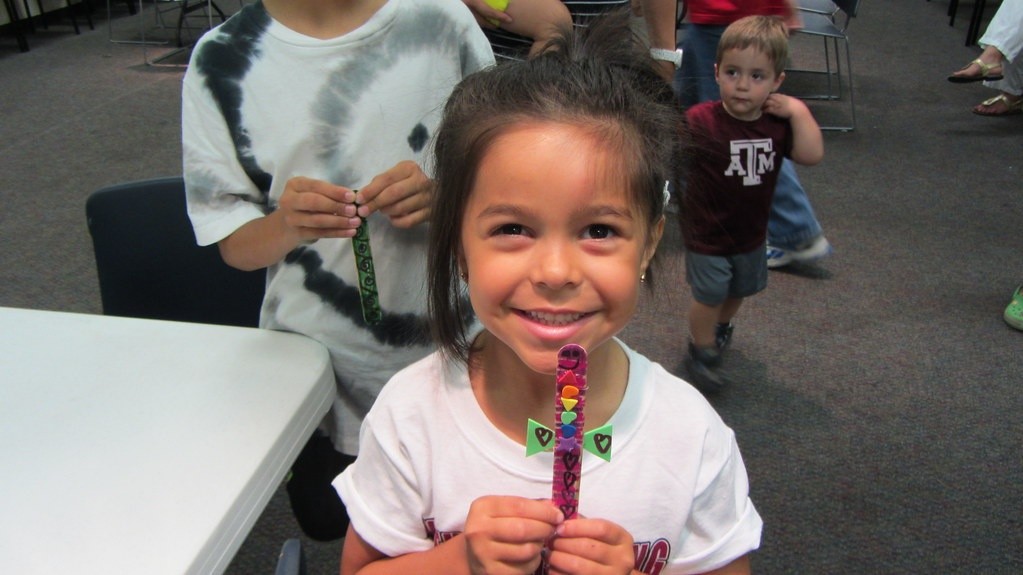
330 9 766 575
463 0 574 58
680 0 833 276
676 15 826 397
182 0 497 542
462 0 684 102
947 0 1023 116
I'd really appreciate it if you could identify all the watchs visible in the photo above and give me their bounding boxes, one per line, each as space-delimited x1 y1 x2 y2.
651 47 683 70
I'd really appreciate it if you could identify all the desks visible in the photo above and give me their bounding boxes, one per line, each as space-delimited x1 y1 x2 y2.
0 306 338 575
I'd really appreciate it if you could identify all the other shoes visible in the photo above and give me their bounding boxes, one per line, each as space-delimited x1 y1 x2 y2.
714 321 734 353
765 234 829 269
687 342 721 379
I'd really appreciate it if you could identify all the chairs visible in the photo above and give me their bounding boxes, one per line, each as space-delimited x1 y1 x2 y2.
86 178 267 328
107 0 243 68
777 0 859 131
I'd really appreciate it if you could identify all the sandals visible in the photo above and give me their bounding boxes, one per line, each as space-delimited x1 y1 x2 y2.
947 58 1004 82
1002 285 1023 330
972 94 1023 117
